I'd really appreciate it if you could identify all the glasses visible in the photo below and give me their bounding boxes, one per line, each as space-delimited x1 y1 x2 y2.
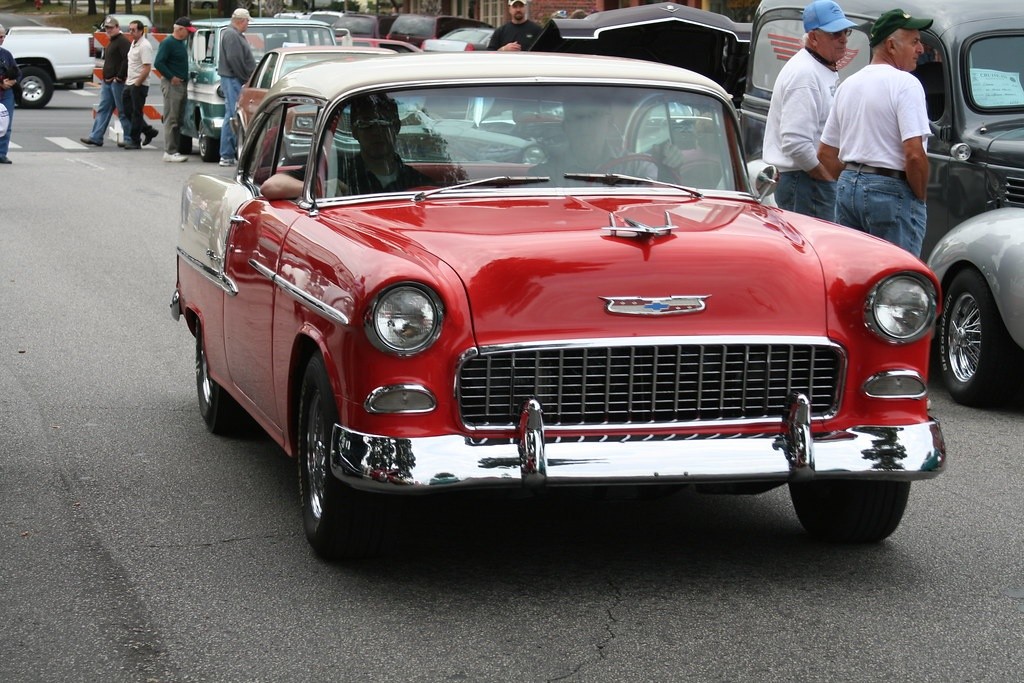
817 29 852 39
354 117 394 129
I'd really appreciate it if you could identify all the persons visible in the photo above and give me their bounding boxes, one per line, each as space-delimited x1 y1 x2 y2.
816 9 934 258
180 183 209 233
80 16 131 147
762 0 857 222
550 10 599 19
218 8 257 167
365 6 399 16
486 0 543 52
0 23 22 164
528 88 726 187
123 20 159 150
154 17 197 162
261 90 437 198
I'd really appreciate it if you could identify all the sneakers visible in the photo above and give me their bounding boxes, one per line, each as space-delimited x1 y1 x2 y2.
219 157 239 167
163 152 188 162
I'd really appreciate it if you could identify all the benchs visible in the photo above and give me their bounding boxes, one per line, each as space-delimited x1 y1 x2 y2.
257 162 537 184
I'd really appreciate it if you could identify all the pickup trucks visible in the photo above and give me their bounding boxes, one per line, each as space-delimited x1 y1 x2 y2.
0 34 95 110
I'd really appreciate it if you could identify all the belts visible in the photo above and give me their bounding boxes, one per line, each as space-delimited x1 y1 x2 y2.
845 162 905 180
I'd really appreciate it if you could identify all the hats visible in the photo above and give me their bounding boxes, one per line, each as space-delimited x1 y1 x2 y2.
869 8 933 47
232 8 256 23
104 18 120 27
175 17 197 32
803 1 859 33
507 0 527 6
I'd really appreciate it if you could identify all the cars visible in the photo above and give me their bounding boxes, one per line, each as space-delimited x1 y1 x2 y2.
6 26 85 90
739 4 1024 412
232 47 422 168
93 14 158 60
181 17 338 163
170 52 948 567
273 12 754 165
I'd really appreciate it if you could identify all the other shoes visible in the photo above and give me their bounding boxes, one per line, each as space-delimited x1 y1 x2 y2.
142 128 159 146
1 155 12 164
124 145 141 149
80 137 103 147
118 142 127 147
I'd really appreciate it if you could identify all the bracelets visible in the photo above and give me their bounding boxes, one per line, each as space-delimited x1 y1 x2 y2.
15 79 17 85
502 46 505 51
